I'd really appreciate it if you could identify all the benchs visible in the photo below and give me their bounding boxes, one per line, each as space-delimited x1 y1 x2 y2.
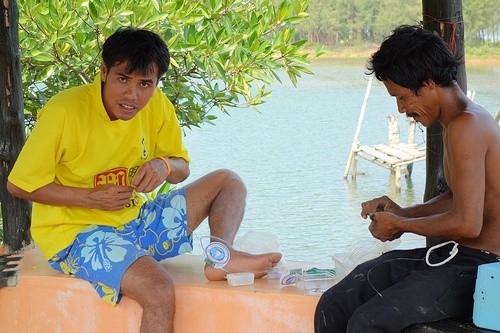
0 250 337 333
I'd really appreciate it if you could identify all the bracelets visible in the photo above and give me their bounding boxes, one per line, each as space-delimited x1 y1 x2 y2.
157 157 171 177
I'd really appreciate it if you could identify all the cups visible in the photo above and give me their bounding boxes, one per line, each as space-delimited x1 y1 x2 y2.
0 256 25 287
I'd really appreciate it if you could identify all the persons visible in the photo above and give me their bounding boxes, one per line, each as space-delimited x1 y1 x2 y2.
7 26 282 333
314 24 500 333
